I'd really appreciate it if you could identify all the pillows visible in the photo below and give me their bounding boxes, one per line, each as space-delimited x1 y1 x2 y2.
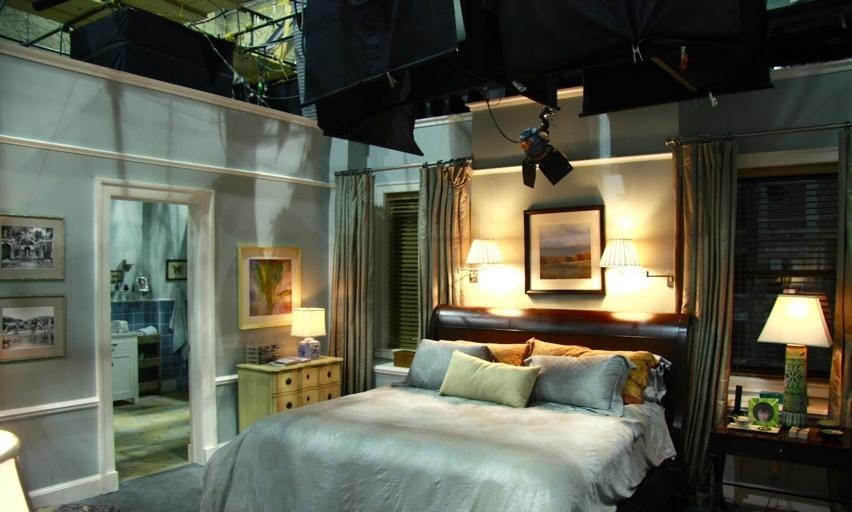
454 339 531 375
526 337 657 402
642 353 672 403
522 353 637 418
438 348 542 408
402 339 493 392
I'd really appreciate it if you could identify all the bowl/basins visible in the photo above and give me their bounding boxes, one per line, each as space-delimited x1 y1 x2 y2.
817 418 840 428
820 428 843 439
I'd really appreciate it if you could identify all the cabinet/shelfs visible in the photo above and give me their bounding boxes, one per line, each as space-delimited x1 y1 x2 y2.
235 355 344 436
112 334 139 404
139 333 162 392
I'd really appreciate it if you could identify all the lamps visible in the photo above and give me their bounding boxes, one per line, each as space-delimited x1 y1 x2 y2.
455 239 505 283
290 307 328 358
596 238 674 290
754 287 833 426
1 429 36 512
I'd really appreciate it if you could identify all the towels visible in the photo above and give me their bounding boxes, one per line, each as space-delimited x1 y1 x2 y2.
138 325 158 336
168 287 187 354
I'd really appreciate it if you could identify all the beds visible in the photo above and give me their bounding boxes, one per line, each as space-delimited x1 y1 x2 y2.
195 302 696 512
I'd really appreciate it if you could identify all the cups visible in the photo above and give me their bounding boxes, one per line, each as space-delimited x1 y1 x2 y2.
734 416 752 427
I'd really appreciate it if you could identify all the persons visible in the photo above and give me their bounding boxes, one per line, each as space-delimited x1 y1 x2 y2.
755 402 774 422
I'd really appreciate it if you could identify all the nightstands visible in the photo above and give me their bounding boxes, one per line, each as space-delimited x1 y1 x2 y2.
374 359 411 388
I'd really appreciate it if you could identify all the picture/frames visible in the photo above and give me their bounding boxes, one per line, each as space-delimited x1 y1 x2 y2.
0 213 66 283
1 295 68 364
166 259 187 280
748 397 780 428
136 276 149 292
523 202 605 295
236 245 302 331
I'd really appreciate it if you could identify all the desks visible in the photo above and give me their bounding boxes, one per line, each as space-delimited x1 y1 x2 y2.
705 413 852 511
